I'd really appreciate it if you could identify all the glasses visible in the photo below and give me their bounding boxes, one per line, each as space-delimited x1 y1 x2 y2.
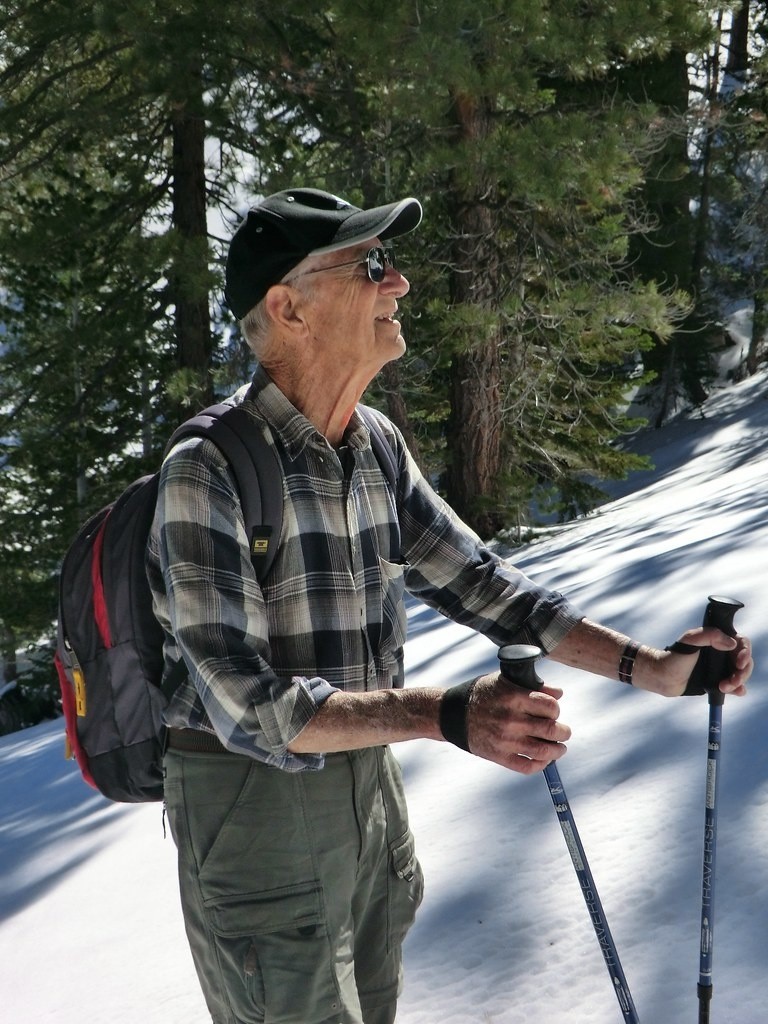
283 245 396 283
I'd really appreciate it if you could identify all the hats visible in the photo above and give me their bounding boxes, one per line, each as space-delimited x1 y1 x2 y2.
224 186 423 320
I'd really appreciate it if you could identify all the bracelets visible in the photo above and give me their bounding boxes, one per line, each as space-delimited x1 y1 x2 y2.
619 639 641 685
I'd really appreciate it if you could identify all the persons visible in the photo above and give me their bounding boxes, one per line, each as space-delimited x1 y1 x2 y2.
146 189 754 1024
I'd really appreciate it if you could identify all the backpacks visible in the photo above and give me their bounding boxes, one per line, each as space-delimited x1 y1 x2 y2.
53 405 285 802
